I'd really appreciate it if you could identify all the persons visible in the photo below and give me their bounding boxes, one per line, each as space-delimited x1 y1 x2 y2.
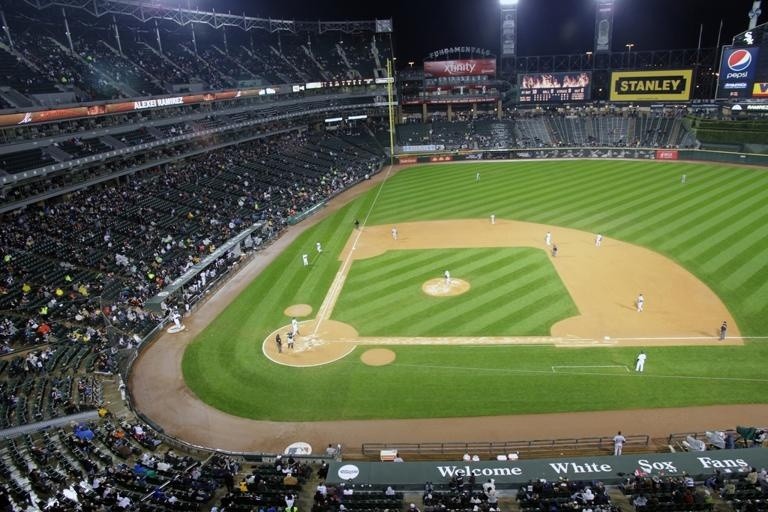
225 431 768 512
680 173 687 184
550 245 559 257
291 317 302 337
443 270 452 285
544 232 553 246
287 332 296 349
592 233 602 246
634 351 647 371
392 102 703 152
474 171 482 184
718 320 727 341
634 294 645 312
391 226 399 241
225 37 387 272
275 334 283 353
489 213 497 225
0 0 226 512
